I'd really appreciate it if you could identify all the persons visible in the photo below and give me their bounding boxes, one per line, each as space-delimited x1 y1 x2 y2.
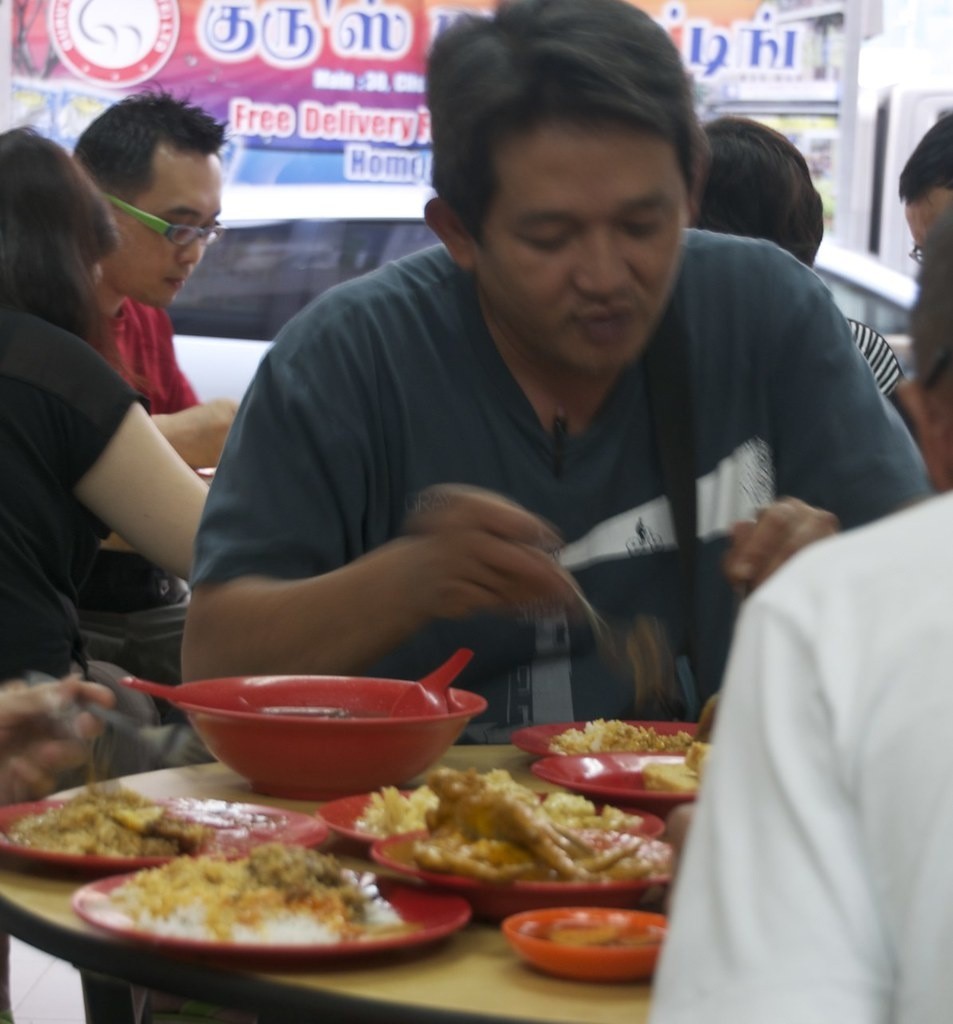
646 108 952 1023
0 82 241 824
179 0 937 742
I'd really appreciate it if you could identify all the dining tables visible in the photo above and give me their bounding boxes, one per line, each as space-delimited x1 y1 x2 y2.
0 743 654 1024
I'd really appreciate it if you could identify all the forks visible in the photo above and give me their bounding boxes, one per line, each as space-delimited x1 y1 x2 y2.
28 673 211 774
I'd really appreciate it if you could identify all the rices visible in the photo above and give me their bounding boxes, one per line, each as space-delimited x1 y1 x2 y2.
547 720 695 755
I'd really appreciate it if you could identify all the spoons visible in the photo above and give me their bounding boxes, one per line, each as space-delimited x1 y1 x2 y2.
115 675 261 712
386 648 474 719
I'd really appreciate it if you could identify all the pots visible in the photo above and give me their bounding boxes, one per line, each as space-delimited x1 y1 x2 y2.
196 467 217 486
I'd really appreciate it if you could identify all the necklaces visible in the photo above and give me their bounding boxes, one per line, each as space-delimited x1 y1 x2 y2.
552 399 573 442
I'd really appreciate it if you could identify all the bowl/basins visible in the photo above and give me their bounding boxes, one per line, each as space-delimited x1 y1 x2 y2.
163 677 488 801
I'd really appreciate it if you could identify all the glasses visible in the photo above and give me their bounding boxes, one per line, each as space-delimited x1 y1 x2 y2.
99 190 225 247
910 244 935 263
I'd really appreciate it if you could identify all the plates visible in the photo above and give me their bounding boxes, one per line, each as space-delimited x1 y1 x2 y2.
315 789 666 841
503 907 671 981
371 836 679 919
530 750 702 807
71 870 472 962
0 798 328 867
509 720 713 759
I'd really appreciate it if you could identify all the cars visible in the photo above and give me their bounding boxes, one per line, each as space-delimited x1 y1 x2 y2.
173 185 920 487
